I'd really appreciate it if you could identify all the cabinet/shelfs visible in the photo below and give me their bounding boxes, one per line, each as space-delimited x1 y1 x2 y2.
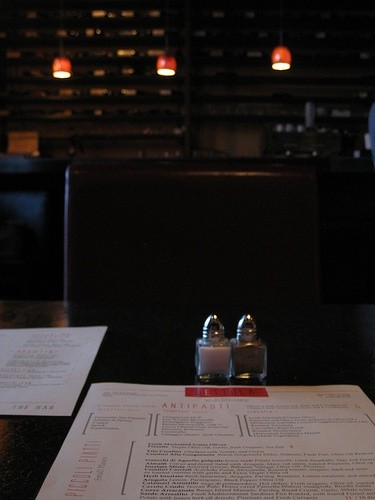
0 0 375 163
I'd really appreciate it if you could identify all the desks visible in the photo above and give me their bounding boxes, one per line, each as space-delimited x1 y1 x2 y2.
0 296 375 500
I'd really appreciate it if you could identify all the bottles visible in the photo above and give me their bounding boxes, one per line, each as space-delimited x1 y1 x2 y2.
229 314 268 380
194 314 233 382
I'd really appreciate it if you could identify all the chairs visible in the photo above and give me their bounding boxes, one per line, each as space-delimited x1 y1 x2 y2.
59 161 324 305
